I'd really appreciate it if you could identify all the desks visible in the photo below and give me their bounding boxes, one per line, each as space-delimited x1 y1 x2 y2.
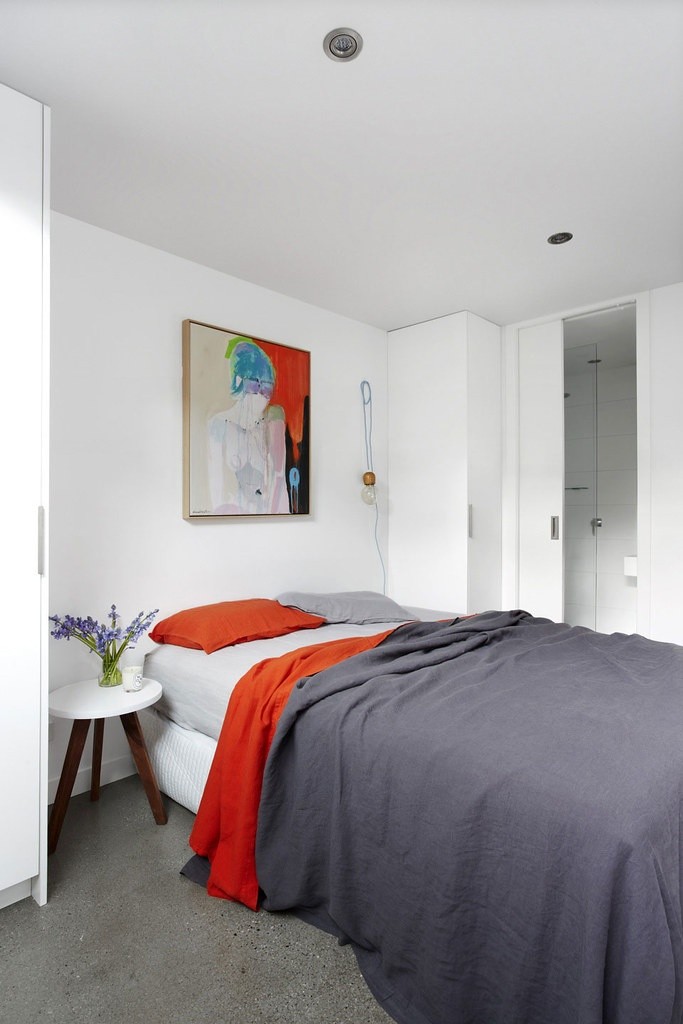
48 677 167 856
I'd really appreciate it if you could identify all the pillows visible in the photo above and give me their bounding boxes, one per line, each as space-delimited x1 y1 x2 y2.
275 591 420 626
147 598 327 655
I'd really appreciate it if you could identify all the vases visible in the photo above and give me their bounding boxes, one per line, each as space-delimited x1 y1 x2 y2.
98 662 123 687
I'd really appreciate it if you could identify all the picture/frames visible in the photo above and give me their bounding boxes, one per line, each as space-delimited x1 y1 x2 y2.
181 319 313 522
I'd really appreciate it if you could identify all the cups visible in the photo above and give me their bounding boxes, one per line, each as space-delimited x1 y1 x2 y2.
122 666 143 692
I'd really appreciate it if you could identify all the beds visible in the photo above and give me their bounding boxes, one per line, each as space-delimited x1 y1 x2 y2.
127 607 683 1024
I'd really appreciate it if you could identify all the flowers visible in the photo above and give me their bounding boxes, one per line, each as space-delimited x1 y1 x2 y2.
49 604 159 663
